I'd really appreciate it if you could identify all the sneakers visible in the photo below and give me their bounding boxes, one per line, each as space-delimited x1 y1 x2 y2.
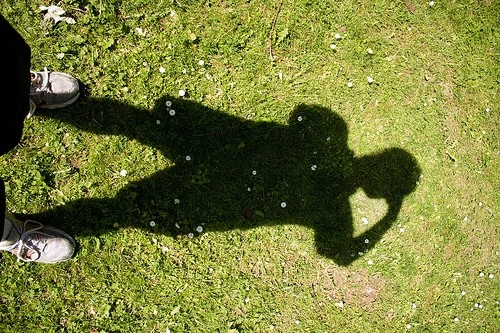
5 213 75 265
27 67 80 110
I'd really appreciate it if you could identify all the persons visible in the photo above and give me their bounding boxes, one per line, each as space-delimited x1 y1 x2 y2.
0 12 81 266
